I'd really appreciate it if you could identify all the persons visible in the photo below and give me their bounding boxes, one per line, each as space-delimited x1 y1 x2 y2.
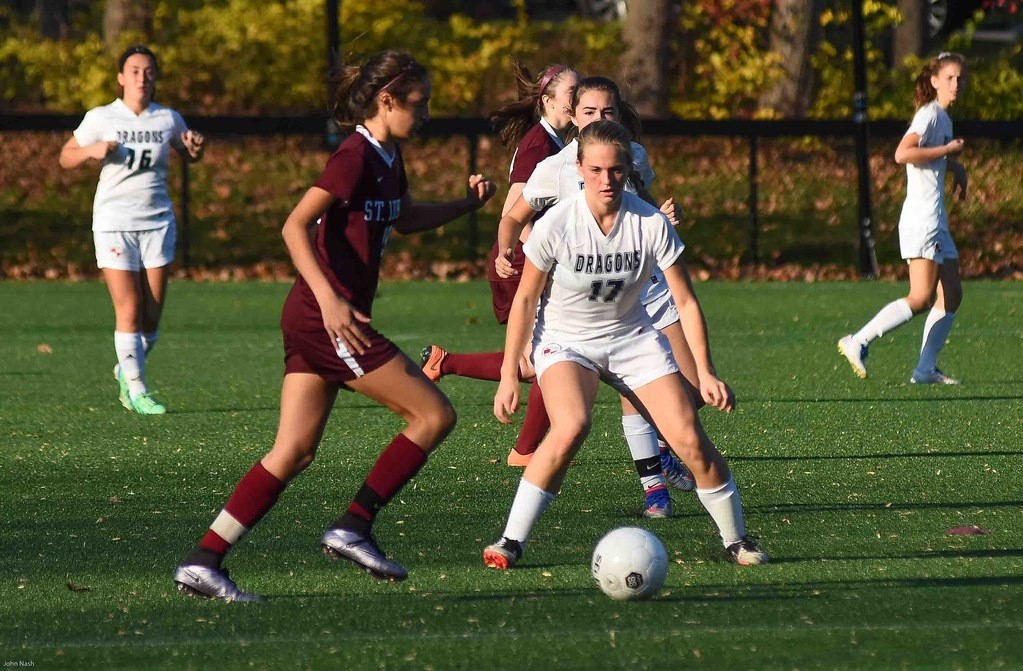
493 77 696 518
58 43 207 413
484 121 766 567
837 51 970 386
415 64 580 467
171 50 497 606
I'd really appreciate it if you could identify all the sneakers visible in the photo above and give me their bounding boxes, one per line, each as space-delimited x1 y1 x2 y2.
113 364 135 410
837 334 869 378
319 527 409 581
419 343 447 382
127 390 166 414
483 537 525 569
173 564 267 603
507 447 534 467
643 486 676 519
910 367 959 384
728 534 769 565
659 451 694 492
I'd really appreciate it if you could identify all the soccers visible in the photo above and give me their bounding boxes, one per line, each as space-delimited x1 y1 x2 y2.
589 525 670 602
